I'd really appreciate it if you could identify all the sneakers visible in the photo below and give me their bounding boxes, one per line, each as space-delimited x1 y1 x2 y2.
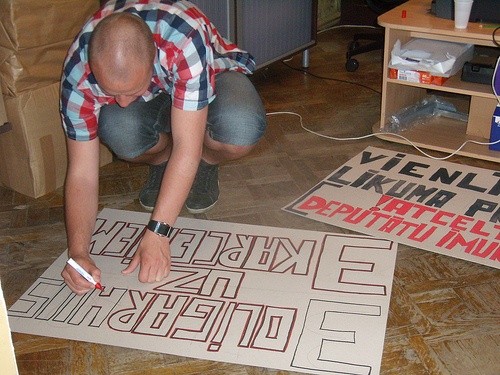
139 158 220 213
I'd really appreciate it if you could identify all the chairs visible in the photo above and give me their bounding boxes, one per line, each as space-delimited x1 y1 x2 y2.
340 0 409 72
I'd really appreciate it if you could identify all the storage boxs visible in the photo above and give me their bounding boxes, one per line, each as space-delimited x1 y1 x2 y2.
0 0 116 199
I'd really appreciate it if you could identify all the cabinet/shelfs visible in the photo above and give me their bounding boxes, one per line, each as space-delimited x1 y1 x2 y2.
373 0 500 162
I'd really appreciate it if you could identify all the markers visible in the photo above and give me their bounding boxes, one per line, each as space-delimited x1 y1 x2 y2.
67 258 104 292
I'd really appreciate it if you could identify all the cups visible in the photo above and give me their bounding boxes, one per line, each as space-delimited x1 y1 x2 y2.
454 0 473 29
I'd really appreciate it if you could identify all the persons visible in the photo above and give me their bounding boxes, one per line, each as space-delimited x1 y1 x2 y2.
58 0 267 295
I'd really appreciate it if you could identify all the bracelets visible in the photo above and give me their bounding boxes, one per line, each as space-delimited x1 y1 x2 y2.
147 219 174 238
